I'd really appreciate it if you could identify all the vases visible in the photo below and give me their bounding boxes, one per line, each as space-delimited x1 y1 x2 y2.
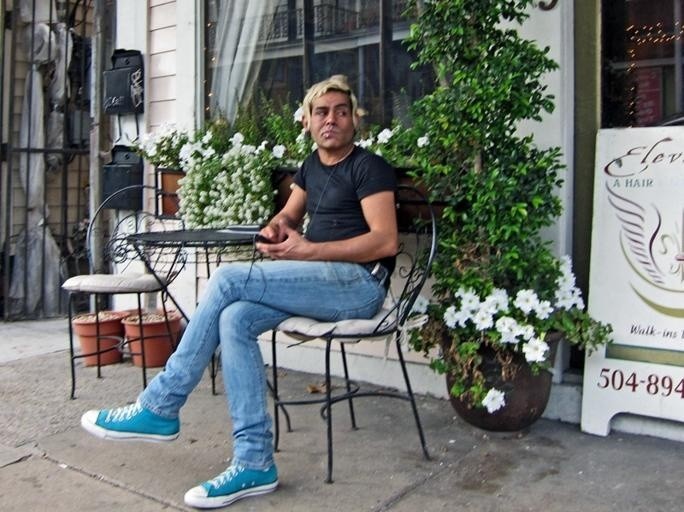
71 310 128 367
445 300 561 433
124 310 183 367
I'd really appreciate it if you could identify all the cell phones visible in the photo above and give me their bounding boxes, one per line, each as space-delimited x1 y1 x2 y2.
257 234 276 244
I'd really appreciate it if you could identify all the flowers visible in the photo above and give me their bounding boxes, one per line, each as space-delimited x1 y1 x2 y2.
405 250 587 414
138 105 431 230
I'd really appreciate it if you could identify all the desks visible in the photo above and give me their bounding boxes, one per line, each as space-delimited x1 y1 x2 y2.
128 224 354 429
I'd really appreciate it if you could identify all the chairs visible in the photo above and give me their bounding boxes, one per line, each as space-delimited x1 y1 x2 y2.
59 184 444 485
61 184 187 398
268 208 443 484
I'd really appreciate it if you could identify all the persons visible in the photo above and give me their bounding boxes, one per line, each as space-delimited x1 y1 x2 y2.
77 73 403 510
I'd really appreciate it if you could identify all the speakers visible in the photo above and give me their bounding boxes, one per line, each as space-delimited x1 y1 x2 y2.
101 50 144 114
97 147 144 210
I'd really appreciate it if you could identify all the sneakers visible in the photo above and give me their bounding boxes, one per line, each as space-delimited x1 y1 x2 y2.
80 399 180 445
184 458 280 509
